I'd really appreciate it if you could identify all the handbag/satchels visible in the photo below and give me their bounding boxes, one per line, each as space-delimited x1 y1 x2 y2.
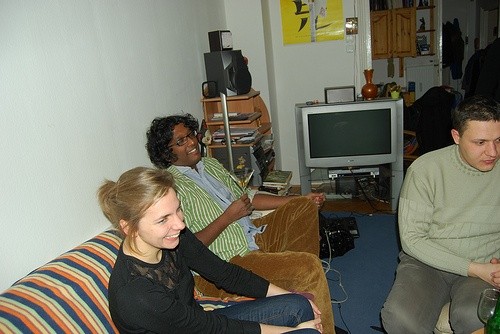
319 222 355 259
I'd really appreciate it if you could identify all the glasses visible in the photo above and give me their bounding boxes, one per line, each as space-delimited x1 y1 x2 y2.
168 128 198 147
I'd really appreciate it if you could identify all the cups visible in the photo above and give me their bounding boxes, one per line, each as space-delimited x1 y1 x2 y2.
390 88 401 100
477 289 500 324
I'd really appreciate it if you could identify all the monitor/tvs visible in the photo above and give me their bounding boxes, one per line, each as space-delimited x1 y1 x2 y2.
301 100 398 167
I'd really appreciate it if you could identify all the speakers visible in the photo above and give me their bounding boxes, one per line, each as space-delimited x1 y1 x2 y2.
203 50 252 96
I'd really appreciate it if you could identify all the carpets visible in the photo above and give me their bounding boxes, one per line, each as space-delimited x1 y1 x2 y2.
317 210 401 334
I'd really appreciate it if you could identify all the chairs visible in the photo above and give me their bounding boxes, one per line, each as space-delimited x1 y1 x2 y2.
404 86 463 160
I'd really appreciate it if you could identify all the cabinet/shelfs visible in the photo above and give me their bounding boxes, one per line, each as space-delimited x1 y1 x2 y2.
369 0 436 59
200 92 276 191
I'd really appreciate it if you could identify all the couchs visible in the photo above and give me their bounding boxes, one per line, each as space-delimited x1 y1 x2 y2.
0 220 255 334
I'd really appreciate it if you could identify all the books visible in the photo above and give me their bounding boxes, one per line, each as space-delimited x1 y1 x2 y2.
258 170 292 196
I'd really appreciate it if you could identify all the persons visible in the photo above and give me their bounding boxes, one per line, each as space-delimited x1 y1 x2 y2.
98 166 323 334
463 38 500 101
381 96 500 334
146 112 336 334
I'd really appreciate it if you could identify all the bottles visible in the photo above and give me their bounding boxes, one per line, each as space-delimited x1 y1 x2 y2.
361 68 378 100
485 293 500 334
